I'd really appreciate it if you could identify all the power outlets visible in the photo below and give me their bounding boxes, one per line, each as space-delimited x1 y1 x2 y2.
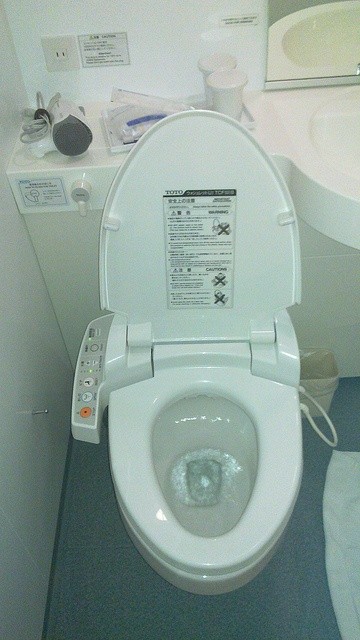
40 35 80 71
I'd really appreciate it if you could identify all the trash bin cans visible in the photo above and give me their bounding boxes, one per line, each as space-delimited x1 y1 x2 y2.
299 348 339 417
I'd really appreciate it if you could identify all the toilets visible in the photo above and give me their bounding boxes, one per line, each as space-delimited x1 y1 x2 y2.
69 109 306 596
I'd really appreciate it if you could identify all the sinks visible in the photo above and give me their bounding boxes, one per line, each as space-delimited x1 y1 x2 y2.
282 9 359 68
309 85 360 188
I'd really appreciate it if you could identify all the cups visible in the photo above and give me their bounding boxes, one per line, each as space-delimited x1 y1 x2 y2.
206 68 248 121
198 52 239 105
